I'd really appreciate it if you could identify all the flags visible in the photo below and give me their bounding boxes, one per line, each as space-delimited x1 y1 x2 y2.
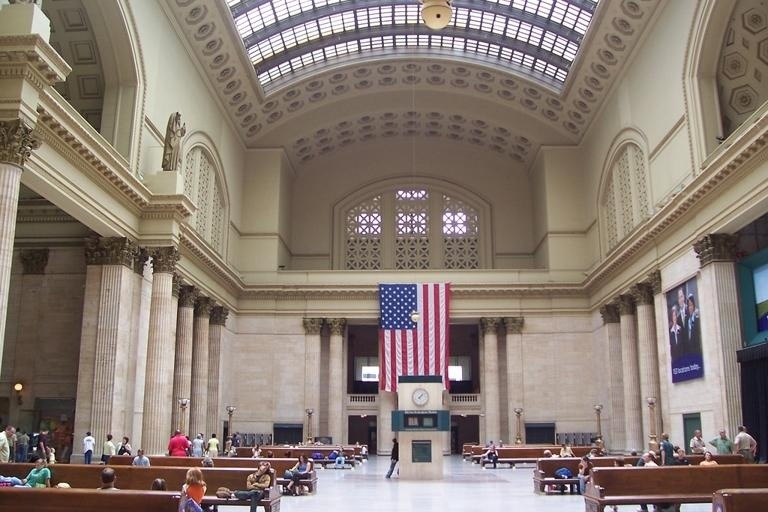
378 283 449 392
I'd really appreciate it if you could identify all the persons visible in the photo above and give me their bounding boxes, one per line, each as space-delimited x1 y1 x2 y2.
168 428 241 468
181 466 207 511
668 306 682 357
152 478 168 491
286 454 311 496
676 287 688 323
116 437 132 456
1 427 56 489
385 437 397 479
101 433 116 464
682 293 699 357
132 449 150 465
268 450 273 458
235 461 271 511
481 443 497 468
83 432 96 464
335 445 346 465
97 467 120 491
251 444 262 458
539 423 756 512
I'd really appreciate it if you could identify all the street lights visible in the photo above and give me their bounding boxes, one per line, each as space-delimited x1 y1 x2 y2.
645 396 661 453
515 407 525 444
225 406 238 439
178 396 191 435
305 407 315 444
593 404 605 439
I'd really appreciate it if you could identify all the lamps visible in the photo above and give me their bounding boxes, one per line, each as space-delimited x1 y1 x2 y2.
13 382 26 405
418 0 453 32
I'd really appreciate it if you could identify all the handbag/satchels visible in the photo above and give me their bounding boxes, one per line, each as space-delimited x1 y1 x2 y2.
215 486 232 499
554 467 573 479
329 450 338 459
283 469 293 479
311 451 325 460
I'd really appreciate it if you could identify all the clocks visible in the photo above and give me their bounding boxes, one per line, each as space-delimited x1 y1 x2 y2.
411 388 430 406
